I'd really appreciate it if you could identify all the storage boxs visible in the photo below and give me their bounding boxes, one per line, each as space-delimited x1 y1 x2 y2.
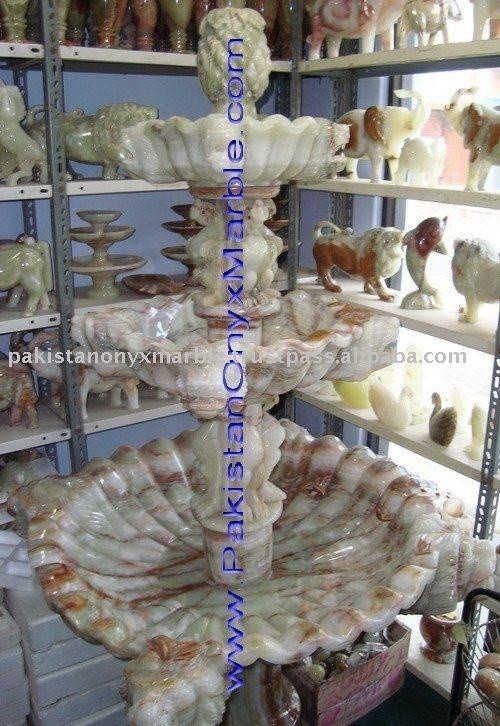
280 617 410 726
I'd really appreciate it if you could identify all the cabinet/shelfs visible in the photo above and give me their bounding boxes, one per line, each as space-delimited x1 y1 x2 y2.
1 0 499 726
